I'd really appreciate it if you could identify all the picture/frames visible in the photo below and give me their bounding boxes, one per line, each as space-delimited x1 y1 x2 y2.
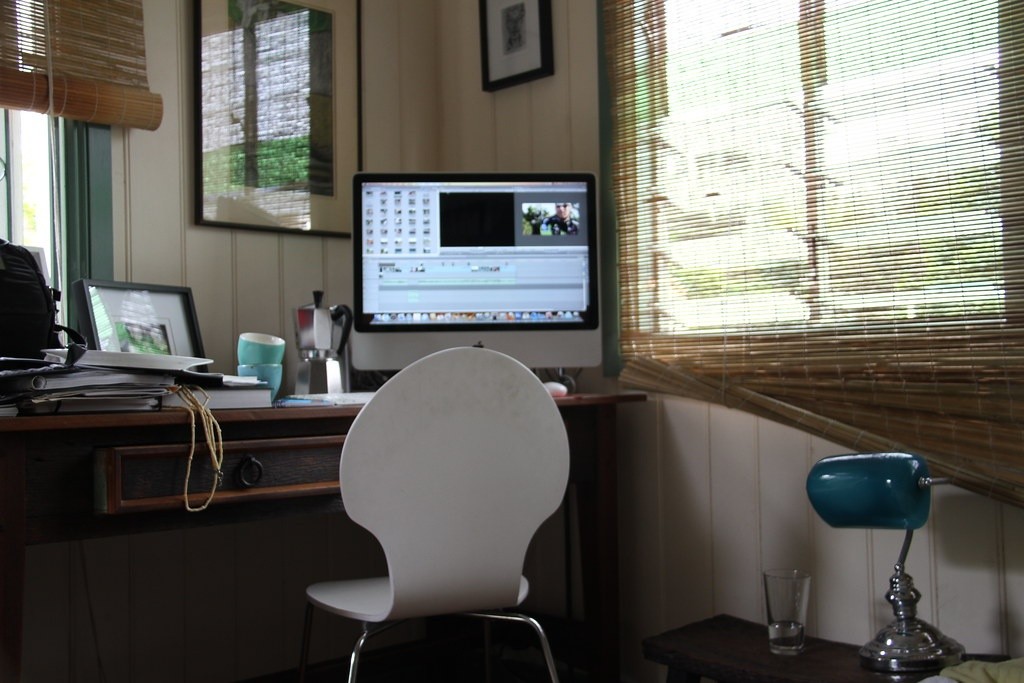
193 0 361 238
71 279 210 373
478 0 553 92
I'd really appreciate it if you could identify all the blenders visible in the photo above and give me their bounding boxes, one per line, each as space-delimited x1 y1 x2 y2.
291 289 353 396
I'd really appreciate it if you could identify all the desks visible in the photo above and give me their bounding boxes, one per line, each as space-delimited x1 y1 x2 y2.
0 392 648 683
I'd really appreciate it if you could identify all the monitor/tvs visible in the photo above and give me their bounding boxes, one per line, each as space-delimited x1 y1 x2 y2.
353 172 605 370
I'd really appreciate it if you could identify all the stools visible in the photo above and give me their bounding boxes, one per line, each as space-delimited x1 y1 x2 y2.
643 614 1009 683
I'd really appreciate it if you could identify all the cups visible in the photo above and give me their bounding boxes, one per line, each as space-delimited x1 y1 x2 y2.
237 333 287 366
763 569 812 655
238 364 284 403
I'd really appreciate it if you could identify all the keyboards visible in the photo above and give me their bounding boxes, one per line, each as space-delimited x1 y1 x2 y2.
288 390 378 407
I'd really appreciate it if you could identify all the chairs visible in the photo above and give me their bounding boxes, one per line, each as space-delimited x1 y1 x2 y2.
299 347 570 683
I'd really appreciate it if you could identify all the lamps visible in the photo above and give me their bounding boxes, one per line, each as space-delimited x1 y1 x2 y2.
807 452 966 672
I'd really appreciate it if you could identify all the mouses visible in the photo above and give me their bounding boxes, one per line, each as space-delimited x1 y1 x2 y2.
544 381 568 396
